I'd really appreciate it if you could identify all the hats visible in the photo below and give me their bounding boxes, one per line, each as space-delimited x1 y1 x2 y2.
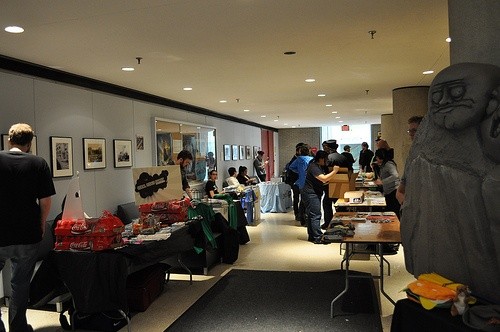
376 136 381 141
326 140 338 150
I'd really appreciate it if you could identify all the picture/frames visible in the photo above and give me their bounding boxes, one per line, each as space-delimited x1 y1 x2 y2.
82 138 106 170
239 145 245 159
1 134 37 156
223 145 232 160
49 136 74 178
245 146 251 160
232 144 238 161
253 146 260 160
113 139 133 168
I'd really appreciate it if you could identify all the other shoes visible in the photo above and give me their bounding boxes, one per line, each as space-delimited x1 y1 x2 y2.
295 216 300 221
27 324 33 332
308 235 330 244
321 223 328 230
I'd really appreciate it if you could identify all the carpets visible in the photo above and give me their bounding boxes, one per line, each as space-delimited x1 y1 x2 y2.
164 269 382 332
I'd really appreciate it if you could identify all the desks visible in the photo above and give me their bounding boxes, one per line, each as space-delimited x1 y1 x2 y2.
190 192 242 263
324 212 403 319
334 190 387 255
28 218 205 332
353 179 379 190
258 181 292 213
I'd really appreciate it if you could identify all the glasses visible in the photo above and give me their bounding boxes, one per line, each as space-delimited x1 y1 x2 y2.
407 129 416 135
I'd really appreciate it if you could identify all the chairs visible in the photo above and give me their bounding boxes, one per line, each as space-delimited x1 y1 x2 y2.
117 202 140 224
44 220 58 245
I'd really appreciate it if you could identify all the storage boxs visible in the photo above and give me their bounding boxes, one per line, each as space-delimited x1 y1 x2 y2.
344 190 364 203
327 167 354 198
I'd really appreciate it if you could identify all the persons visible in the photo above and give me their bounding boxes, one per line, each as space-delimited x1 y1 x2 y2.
237 166 258 187
226 167 242 190
205 171 226 199
284 139 357 245
165 149 194 202
396 114 425 207
253 150 269 182
358 142 374 173
401 62 500 304
0 123 57 332
206 152 216 174
369 139 402 224
